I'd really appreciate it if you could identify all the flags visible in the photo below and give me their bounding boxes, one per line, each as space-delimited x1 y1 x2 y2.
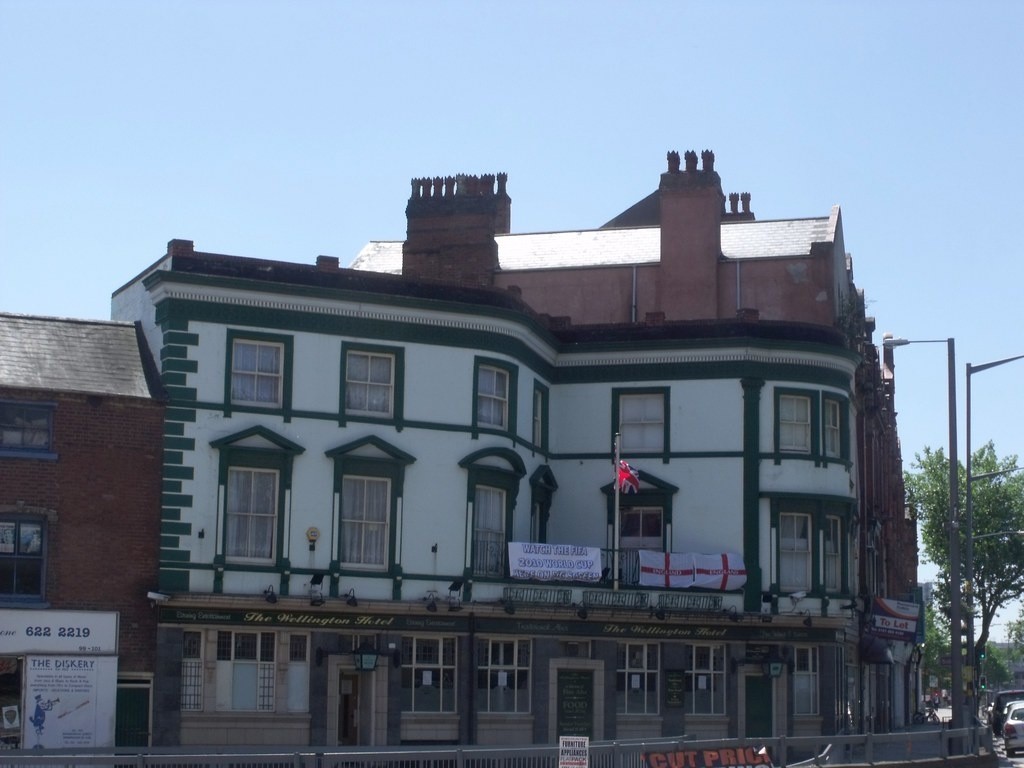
614 459 640 496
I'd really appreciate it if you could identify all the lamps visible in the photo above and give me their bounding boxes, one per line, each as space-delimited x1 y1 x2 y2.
354 637 379 671
760 645 784 679
722 604 744 623
448 597 463 612
423 593 437 613
648 602 666 621
264 585 278 603
308 590 325 607
499 597 518 614
345 588 357 607
572 602 587 619
801 609 813 627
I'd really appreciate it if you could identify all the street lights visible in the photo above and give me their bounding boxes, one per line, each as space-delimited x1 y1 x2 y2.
883 338 963 759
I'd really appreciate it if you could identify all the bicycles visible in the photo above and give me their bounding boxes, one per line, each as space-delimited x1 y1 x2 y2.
914 707 940 725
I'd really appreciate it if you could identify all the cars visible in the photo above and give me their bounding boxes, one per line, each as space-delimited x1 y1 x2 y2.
1001 701 1024 732
1000 703 1024 758
985 702 995 725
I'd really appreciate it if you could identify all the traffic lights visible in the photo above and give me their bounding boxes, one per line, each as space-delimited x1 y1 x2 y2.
979 676 986 689
979 645 987 661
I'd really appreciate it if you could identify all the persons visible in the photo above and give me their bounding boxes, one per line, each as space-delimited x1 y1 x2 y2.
933 693 941 711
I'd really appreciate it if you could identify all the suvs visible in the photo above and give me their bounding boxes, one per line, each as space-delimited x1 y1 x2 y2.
988 690 1024 736
